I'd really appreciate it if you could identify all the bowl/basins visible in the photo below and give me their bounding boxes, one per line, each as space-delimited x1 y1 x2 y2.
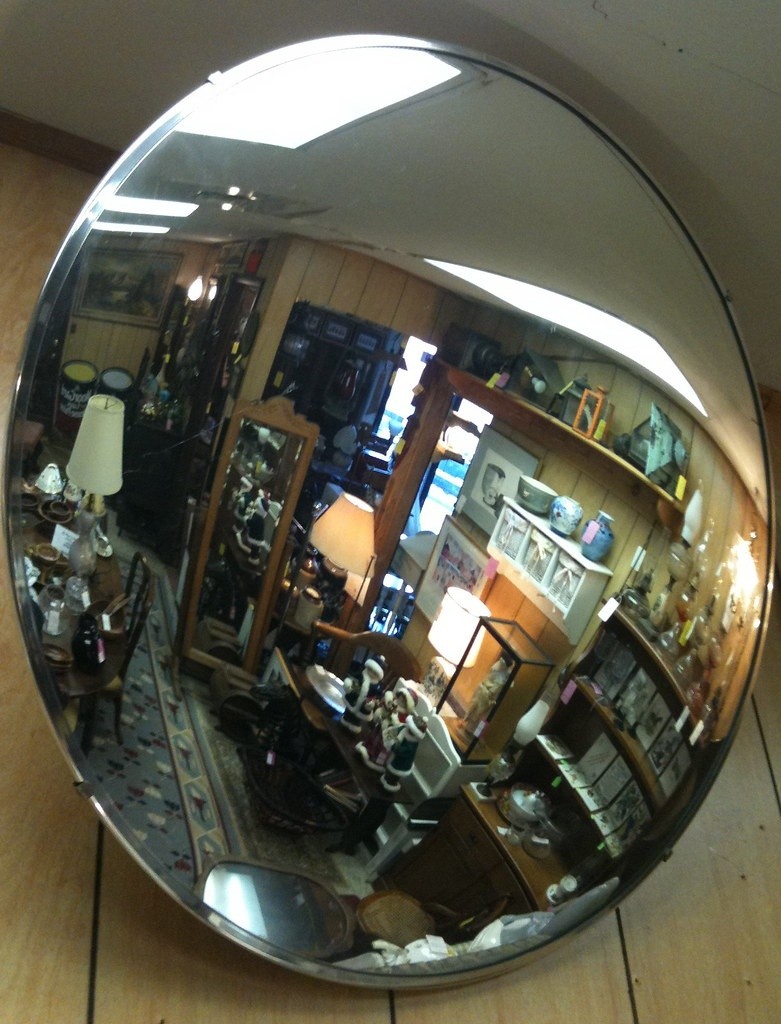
86 600 125 640
515 475 558 515
509 782 553 823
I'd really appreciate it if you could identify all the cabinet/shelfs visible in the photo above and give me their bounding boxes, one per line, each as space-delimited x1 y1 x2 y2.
260 297 403 464
322 712 416 856
444 360 687 535
207 620 424 829
485 493 614 646
376 600 721 945
355 675 493 880
277 587 341 661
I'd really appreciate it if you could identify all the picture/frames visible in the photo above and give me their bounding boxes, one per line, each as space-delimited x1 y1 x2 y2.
456 423 543 536
71 246 186 330
410 516 499 627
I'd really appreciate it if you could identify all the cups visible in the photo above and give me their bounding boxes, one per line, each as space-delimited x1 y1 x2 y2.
506 816 530 847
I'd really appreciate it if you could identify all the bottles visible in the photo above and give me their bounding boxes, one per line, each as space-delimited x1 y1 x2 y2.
581 511 615 563
548 496 584 538
294 585 324 631
71 612 101 667
36 584 71 636
274 577 299 618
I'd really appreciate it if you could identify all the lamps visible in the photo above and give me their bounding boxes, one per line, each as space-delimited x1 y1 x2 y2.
187 270 206 301
424 256 709 421
176 46 487 157
90 220 171 236
425 586 492 697
308 491 377 607
67 391 126 534
105 195 200 219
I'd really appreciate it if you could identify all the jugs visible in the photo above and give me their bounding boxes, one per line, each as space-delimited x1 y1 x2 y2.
522 805 582 861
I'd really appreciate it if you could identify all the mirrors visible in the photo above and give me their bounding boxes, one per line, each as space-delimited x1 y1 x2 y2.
0 27 781 991
171 395 318 679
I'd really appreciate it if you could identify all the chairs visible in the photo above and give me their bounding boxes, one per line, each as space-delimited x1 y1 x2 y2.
81 552 157 757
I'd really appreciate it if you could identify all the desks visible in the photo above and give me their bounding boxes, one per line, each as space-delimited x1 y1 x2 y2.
18 472 128 692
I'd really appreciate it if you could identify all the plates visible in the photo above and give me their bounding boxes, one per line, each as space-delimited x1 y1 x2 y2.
495 788 525 829
